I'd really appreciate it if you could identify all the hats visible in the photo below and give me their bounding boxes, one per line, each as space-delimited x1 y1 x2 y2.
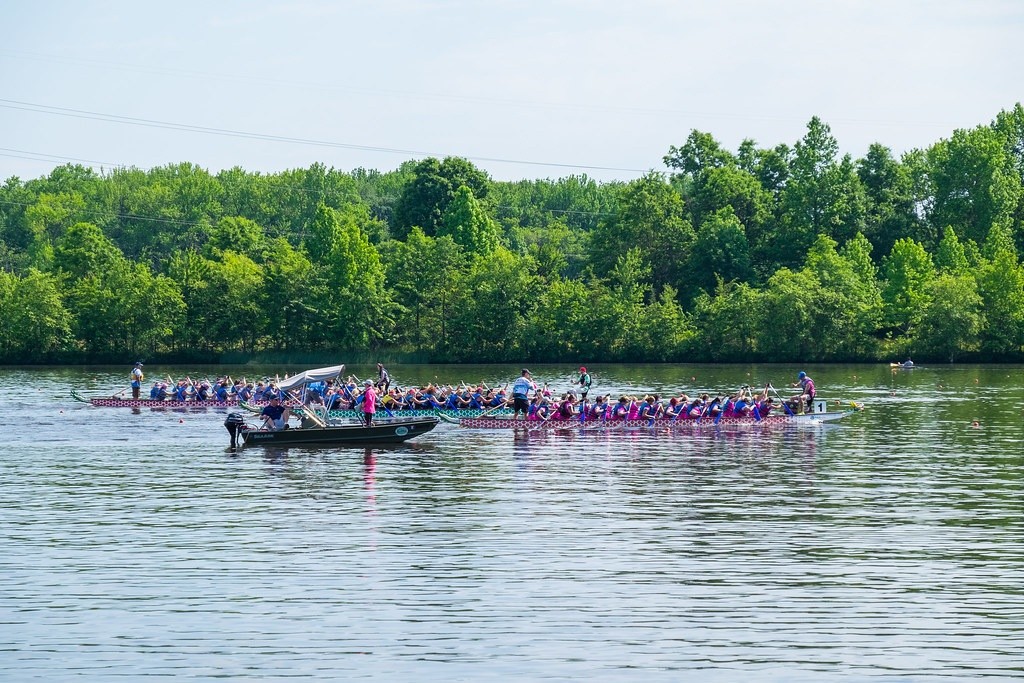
363 379 373 386
477 387 483 393
409 389 414 394
522 368 531 374
378 363 383 368
578 367 586 373
162 384 167 387
501 391 506 394
544 391 552 395
136 361 143 366
670 398 678 402
798 371 806 379
203 383 209 387
339 390 344 394
269 395 279 399
389 389 394 393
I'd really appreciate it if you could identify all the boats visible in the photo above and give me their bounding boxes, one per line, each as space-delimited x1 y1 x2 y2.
238 400 524 417
224 364 442 446
90 398 303 408
458 394 856 431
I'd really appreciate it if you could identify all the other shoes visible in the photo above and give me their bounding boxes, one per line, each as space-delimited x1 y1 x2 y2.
797 412 805 415
284 424 289 430
268 426 278 431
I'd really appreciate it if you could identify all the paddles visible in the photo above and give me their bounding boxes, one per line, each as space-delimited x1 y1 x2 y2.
697 406 707 424
747 387 761 421
437 386 462 416
603 397 610 425
416 389 444 414
533 410 557 431
647 404 661 428
669 402 686 427
715 399 726 425
482 380 505 413
581 400 585 425
187 376 213 410
461 382 483 413
397 388 418 416
768 383 795 417
624 398 634 425
344 384 359 405
375 394 394 417
229 377 245 403
167 374 191 411
206 379 233 411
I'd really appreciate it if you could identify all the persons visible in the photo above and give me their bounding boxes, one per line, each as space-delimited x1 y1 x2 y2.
150 372 309 405
130 362 144 399
512 368 537 421
260 394 290 430
374 362 391 396
361 379 375 427
790 371 816 416
526 382 783 421
567 367 590 402
904 357 914 367
306 376 515 410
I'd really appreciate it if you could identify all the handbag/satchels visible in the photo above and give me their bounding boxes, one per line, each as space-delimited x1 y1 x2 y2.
131 379 141 389
355 402 362 413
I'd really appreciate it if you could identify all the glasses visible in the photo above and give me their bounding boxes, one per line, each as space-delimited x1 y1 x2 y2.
275 399 278 401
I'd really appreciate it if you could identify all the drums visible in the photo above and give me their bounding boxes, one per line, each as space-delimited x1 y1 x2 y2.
784 400 799 415
561 392 568 401
